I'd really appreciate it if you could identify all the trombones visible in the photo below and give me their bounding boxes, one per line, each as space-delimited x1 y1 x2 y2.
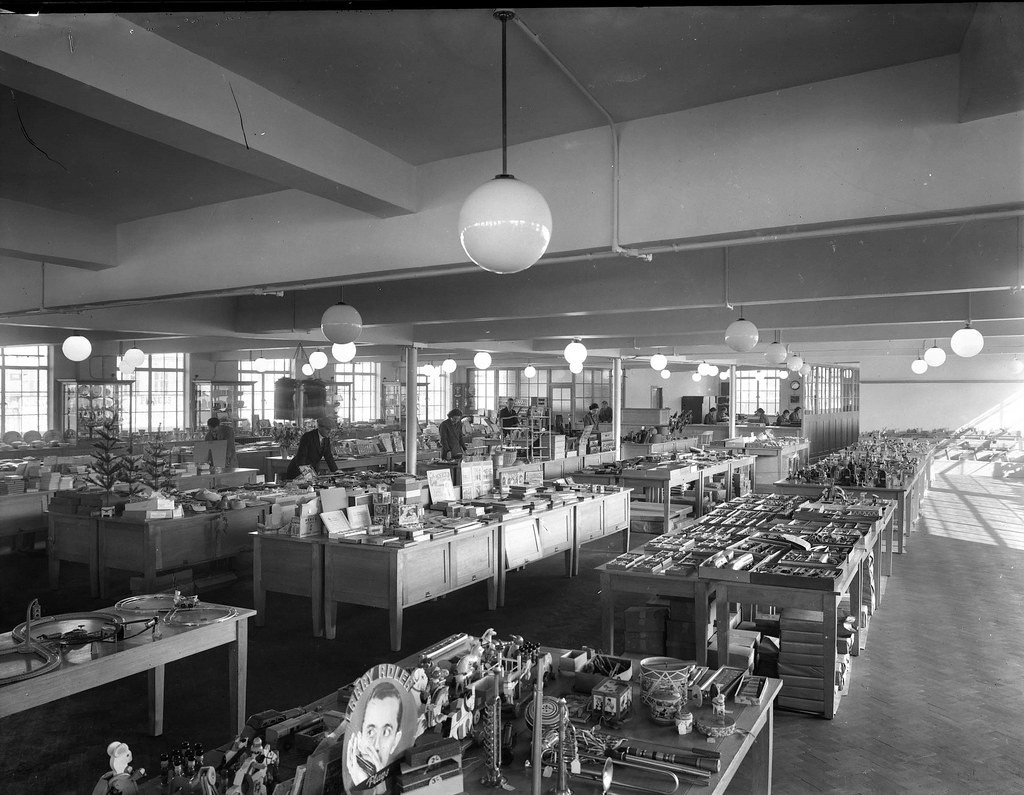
524 746 678 794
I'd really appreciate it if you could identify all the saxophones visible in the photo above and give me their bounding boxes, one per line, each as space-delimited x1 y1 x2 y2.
478 665 508 789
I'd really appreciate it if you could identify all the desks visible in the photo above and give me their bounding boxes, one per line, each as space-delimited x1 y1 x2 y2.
771 444 934 554
877 500 899 605
0 602 257 739
621 437 699 460
569 464 620 485
0 430 634 651
132 636 783 795
711 561 861 720
595 534 717 667
729 455 758 499
852 531 880 609
700 462 730 516
704 441 812 481
618 470 702 533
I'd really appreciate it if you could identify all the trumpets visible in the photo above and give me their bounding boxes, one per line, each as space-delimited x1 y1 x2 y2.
548 697 574 795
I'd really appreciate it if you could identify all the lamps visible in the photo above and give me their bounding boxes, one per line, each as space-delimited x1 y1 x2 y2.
724 305 758 353
301 342 356 376
924 339 946 367
911 350 928 374
119 339 144 375
442 354 457 373
763 330 786 364
459 8 554 273
950 292 984 358
321 287 362 344
1007 354 1024 374
787 352 811 374
62 330 92 361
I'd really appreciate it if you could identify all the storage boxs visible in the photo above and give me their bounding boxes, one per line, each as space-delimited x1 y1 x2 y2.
735 674 769 706
631 473 750 532
558 648 634 720
291 515 321 538
606 492 890 591
130 572 174 590
123 510 172 520
174 567 193 583
125 498 174 510
620 595 868 713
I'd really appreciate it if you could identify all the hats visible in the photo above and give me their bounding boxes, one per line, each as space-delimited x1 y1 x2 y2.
317 418 334 429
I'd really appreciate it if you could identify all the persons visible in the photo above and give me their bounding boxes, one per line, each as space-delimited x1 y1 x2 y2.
757 408 769 426
205 418 240 468
716 407 729 422
438 408 468 465
286 416 345 482
346 682 403 795
776 410 792 426
499 398 520 437
789 407 801 422
704 407 718 425
599 401 612 423
583 404 602 430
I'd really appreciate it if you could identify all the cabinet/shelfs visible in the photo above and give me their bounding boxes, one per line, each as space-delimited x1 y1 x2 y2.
500 406 552 463
453 383 478 416
56 377 136 446
381 374 430 425
192 379 258 432
